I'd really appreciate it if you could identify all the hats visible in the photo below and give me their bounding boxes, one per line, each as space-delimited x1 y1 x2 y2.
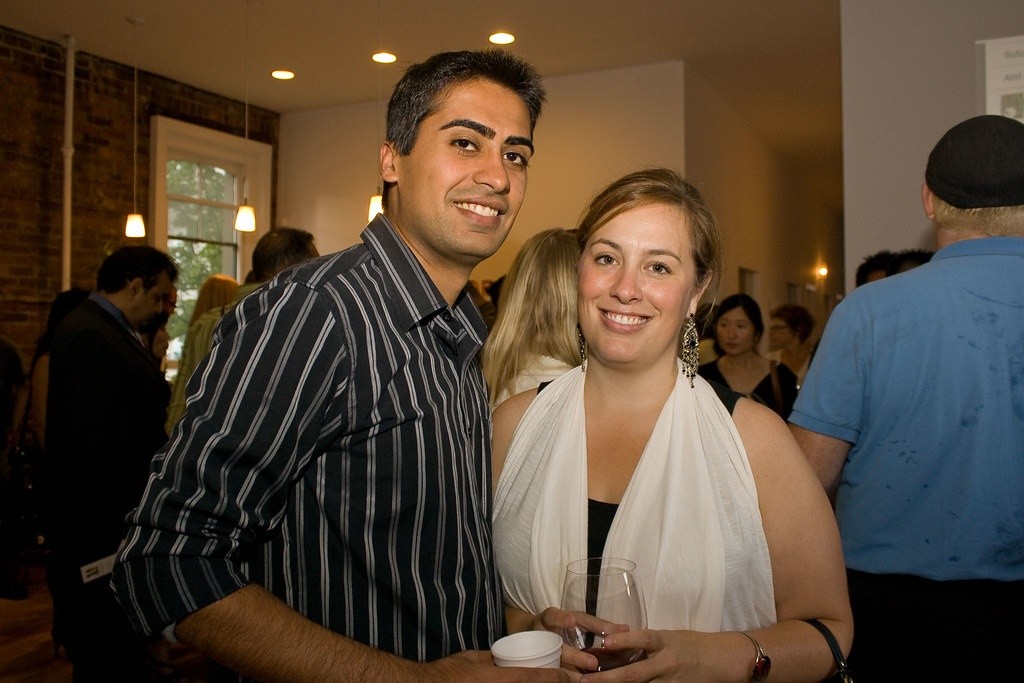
925 115 1024 209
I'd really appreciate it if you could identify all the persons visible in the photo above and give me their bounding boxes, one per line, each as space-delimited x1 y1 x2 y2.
788 115 1024 683
490 168 855 683
1 229 317 683
699 249 934 426
465 226 585 409
110 48 569 683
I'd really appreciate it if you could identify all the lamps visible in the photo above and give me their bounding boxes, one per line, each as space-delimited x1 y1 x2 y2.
368 15 384 222
126 14 146 237
235 21 256 231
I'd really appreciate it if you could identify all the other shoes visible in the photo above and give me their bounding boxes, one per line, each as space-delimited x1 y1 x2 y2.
1 582 28 599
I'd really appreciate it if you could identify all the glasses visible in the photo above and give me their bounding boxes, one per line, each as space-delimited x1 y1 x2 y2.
770 325 789 333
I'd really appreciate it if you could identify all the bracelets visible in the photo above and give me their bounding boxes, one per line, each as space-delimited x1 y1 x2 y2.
736 630 770 683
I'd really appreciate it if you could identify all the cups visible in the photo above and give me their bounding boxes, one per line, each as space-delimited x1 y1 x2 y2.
489 630 564 670
561 557 649 675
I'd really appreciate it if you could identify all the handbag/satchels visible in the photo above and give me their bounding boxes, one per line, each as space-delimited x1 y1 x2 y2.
8 445 43 484
